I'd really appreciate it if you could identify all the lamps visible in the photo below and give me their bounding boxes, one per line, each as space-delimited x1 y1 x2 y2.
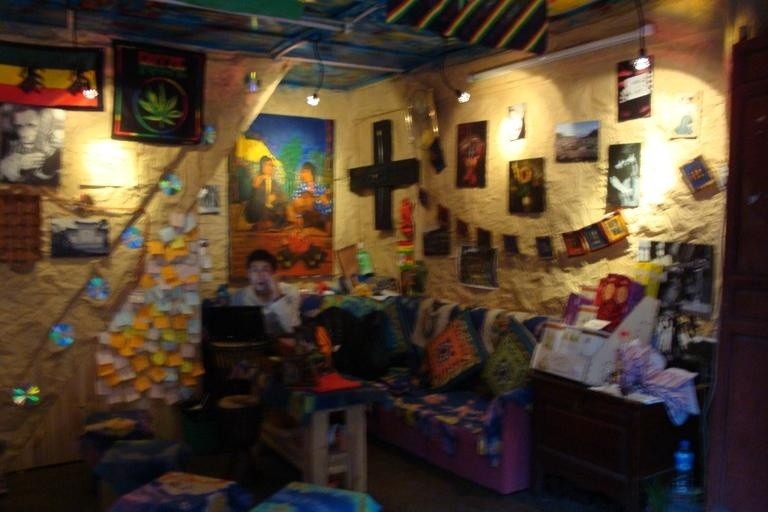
439 47 471 104
76 65 99 99
306 36 325 106
631 1 651 71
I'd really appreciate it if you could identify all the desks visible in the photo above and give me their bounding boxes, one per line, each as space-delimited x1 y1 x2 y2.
264 372 386 489
171 339 290 482
527 368 711 512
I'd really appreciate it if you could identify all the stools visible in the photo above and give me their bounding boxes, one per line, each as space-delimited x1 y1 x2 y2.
83 408 158 504
246 479 386 512
109 468 249 512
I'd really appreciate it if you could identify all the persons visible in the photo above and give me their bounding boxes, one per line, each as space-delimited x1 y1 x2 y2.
230 247 302 334
236 153 290 230
608 152 635 207
1 102 61 188
285 160 333 232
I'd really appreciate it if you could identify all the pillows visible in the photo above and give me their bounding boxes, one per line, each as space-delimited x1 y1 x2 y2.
384 299 414 355
426 306 483 394
478 314 539 395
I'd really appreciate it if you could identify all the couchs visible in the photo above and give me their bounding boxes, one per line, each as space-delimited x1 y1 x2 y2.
291 291 549 495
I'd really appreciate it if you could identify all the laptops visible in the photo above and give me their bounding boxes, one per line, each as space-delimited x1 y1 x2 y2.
200 304 264 342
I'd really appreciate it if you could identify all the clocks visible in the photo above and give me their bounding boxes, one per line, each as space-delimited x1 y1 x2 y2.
404 83 440 151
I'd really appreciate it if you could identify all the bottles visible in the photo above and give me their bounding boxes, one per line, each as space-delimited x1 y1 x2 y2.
671 441 692 495
